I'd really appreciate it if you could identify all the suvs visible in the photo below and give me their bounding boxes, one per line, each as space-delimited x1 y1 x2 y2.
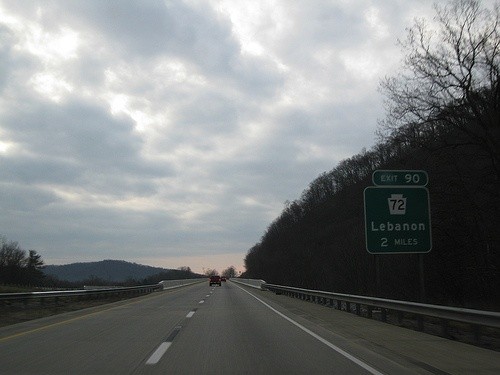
208 275 221 286
220 277 226 282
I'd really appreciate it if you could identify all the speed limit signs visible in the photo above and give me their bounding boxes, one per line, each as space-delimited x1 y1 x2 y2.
363 185 433 253
